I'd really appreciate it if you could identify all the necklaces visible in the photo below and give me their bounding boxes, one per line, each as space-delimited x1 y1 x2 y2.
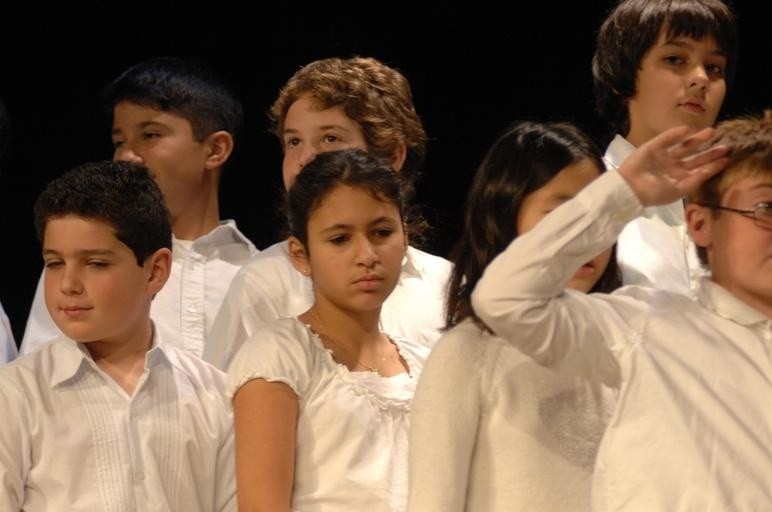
307 310 386 370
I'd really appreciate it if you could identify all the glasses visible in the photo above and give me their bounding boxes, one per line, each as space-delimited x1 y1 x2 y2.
718 206 770 230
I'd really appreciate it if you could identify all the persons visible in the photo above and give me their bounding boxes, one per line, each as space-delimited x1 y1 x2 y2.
403 123 622 510
22 60 259 371
229 147 432 508
472 114 770 512
203 56 455 362
592 0 732 295
2 161 241 511
